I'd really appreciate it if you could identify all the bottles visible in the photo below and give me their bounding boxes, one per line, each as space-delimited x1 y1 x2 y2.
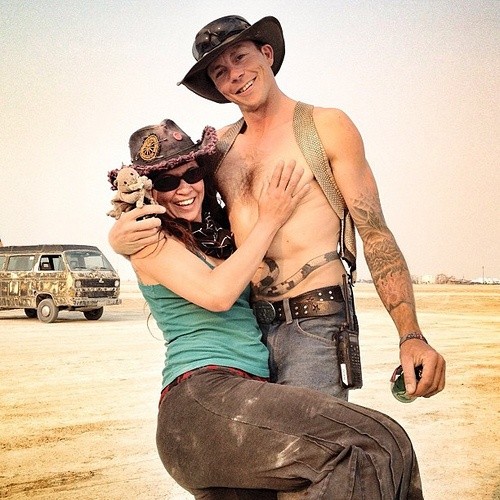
392 364 423 403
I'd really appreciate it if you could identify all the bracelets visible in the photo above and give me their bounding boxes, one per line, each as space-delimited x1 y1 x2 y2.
400 331 425 346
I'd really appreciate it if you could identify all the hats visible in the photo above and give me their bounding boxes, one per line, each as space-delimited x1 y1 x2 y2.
108 117 218 190
176 14 286 105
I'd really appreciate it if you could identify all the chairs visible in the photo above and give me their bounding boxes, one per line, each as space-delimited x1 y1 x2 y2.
71 260 78 266
40 261 52 271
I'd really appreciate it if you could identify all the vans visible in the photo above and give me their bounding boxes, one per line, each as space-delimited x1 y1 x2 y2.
0 244 123 324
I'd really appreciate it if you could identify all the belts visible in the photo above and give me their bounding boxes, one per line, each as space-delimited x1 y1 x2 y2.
249 285 347 325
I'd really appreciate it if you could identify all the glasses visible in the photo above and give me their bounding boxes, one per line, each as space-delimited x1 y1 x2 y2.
153 166 206 192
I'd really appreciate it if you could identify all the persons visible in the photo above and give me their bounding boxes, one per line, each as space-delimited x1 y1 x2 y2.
106 119 424 500
108 15 452 405
